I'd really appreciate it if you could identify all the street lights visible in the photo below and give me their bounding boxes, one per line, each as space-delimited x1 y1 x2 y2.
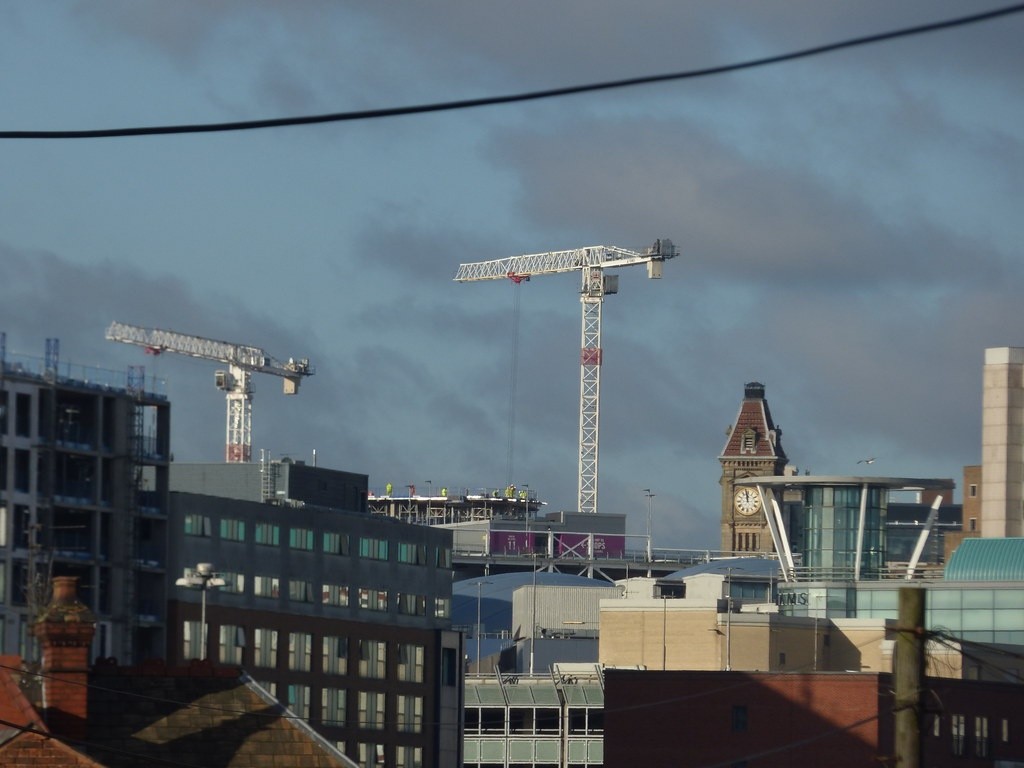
175 563 232 661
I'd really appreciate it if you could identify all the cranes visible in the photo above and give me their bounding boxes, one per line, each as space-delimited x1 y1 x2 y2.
104 320 316 464
452 238 679 514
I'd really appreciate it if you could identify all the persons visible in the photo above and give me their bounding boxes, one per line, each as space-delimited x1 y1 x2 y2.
386 482 393 497
505 484 516 497
410 482 415 496
442 488 448 496
519 491 527 500
492 488 501 498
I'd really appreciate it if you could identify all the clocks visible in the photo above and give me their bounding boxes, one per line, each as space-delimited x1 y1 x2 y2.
733 486 762 515
